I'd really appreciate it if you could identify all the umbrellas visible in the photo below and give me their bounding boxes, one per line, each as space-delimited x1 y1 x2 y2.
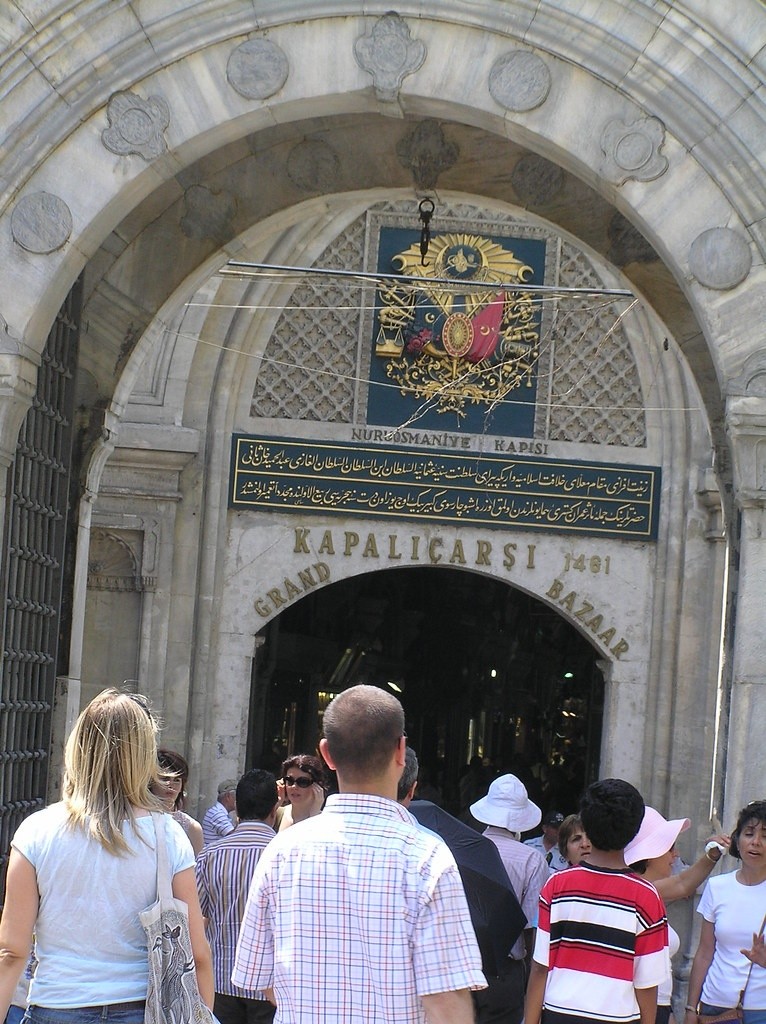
410 799 528 976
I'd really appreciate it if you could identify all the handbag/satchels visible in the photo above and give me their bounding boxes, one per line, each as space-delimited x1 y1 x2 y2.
697 1007 743 1024
138 810 221 1024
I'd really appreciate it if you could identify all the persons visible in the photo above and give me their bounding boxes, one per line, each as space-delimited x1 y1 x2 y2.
523 777 670 1024
684 799 766 1024
203 778 238 846
273 755 326 836
0 688 215 1024
231 684 488 1024
623 805 731 1024
195 769 280 1024
151 750 204 856
468 774 591 1024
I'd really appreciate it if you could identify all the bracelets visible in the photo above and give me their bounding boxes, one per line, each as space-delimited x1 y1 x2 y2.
706 854 717 863
685 1006 696 1012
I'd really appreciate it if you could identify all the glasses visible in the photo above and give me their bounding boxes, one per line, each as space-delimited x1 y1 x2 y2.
283 775 314 788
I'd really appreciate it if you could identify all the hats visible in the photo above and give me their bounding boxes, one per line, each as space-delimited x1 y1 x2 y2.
469 773 542 832
544 811 566 825
218 779 238 792
624 805 691 867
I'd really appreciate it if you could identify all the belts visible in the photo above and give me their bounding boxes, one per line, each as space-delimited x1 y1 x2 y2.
83 1000 146 1011
